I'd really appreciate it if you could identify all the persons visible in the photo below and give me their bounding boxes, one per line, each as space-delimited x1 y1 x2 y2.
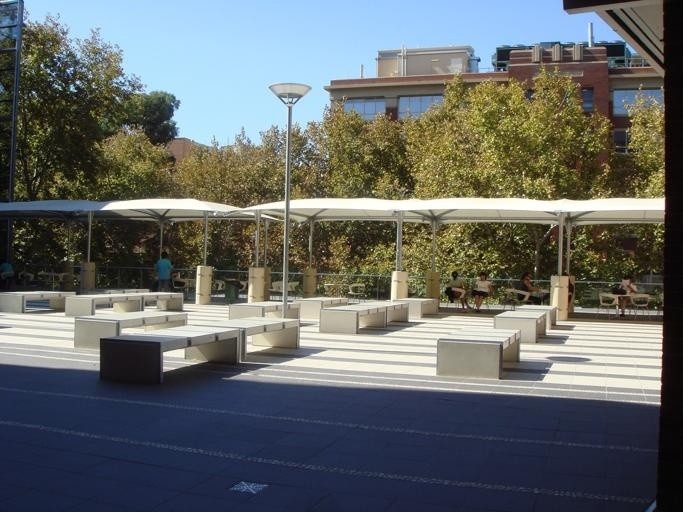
471 271 493 312
0 258 15 286
447 271 472 313
517 272 541 305
562 271 574 304
617 275 637 319
155 252 173 292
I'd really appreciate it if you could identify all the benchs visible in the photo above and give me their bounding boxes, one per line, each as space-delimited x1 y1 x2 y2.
185 317 300 362
228 301 301 319
99 324 241 382
298 297 438 335
495 310 546 344
74 312 188 349
436 329 520 380
1 288 183 316
517 304 555 331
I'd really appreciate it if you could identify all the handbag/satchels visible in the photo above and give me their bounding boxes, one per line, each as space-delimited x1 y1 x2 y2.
613 284 627 295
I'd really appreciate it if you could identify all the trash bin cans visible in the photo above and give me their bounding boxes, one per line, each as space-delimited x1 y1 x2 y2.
223 280 239 300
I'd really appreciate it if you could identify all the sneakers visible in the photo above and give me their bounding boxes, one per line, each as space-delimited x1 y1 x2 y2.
620 314 624 318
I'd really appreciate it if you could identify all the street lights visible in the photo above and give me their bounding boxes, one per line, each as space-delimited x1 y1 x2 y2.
268 82 311 319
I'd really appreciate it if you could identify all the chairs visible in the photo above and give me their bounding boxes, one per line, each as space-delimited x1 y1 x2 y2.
596 292 649 320
171 277 366 303
444 287 555 314
